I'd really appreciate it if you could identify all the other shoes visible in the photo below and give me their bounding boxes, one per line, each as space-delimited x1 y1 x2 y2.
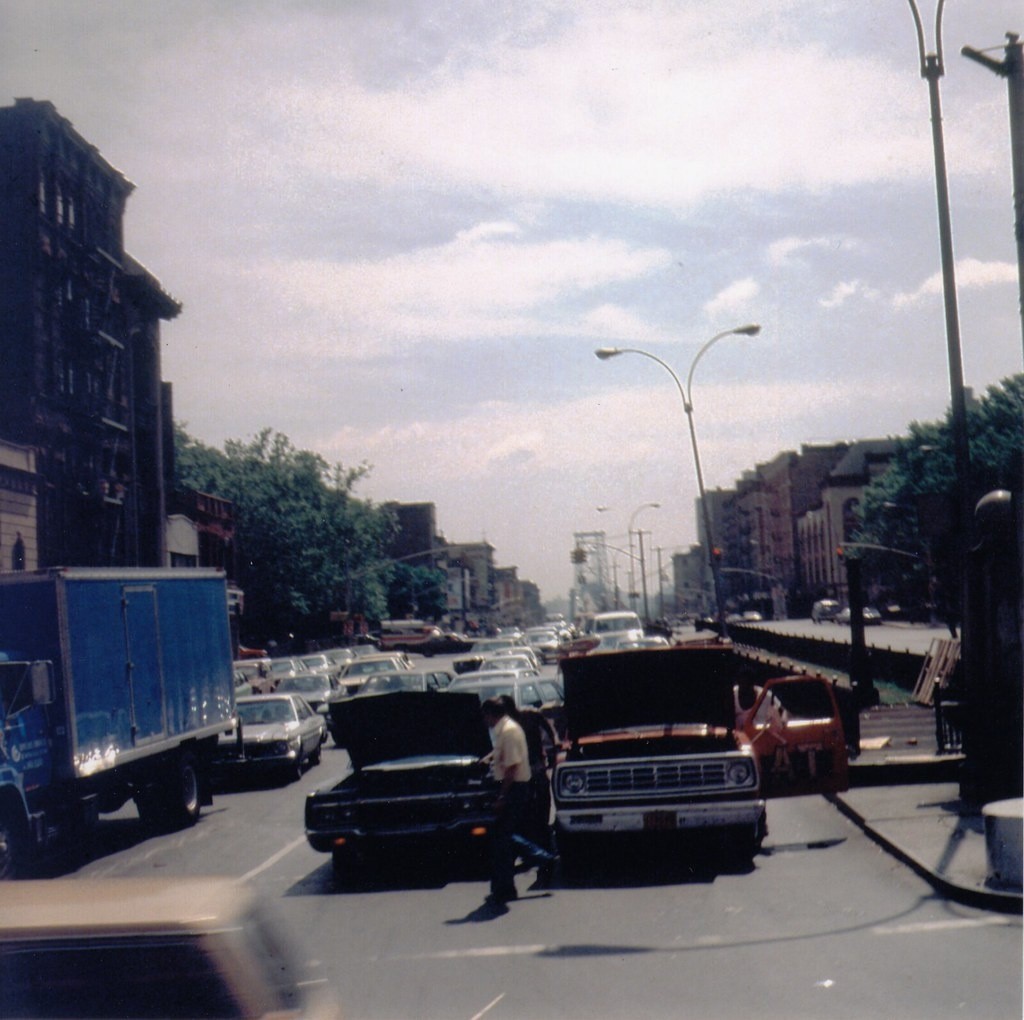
537 854 562 885
485 888 520 902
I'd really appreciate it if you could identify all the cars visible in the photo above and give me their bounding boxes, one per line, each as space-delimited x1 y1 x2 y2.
297 685 542 869
0 876 345 1020
550 641 772 873
811 598 885 628
202 603 652 791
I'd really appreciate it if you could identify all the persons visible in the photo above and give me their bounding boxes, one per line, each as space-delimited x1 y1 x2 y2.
481 695 562 903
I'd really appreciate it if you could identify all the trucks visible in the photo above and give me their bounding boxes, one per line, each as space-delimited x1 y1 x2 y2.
0 566 237 882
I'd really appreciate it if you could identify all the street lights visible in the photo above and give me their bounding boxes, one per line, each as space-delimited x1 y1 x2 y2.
592 322 762 640
594 501 662 633
